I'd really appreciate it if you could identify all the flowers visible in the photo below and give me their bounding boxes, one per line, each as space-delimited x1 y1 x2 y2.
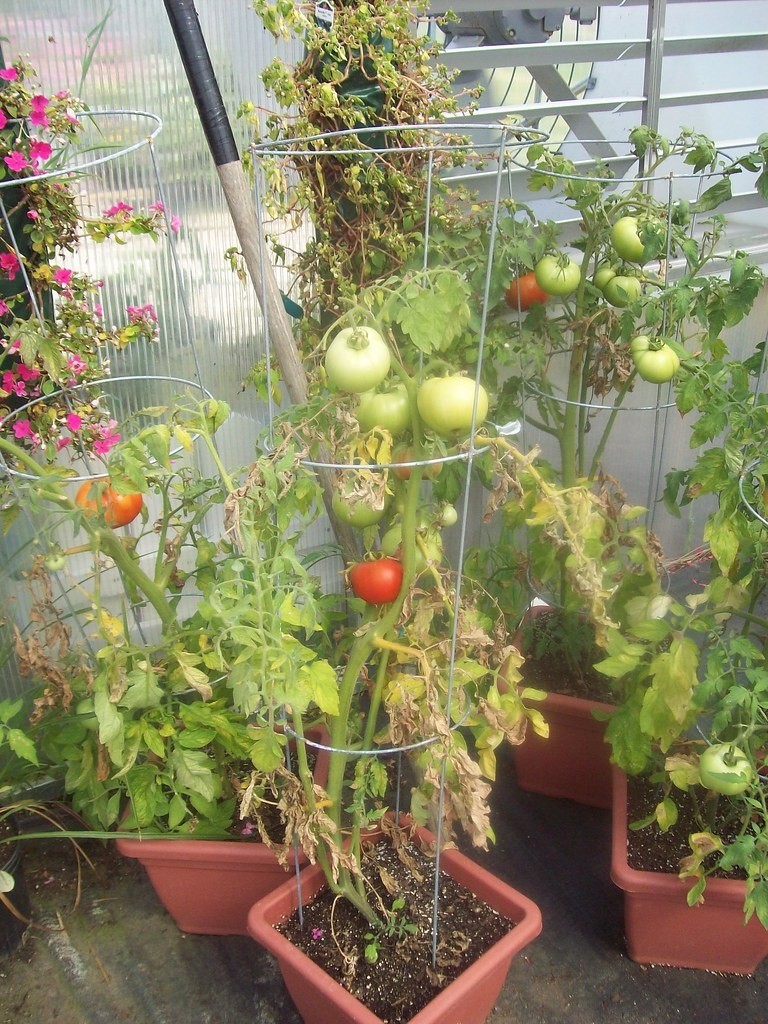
0 0 179 455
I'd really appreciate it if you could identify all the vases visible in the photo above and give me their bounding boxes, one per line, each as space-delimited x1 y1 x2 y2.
116 718 338 933
496 606 614 811
249 812 543 1024
613 742 768 978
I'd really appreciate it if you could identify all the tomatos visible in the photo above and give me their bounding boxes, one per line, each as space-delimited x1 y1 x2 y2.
699 743 752 795
74 213 680 602
